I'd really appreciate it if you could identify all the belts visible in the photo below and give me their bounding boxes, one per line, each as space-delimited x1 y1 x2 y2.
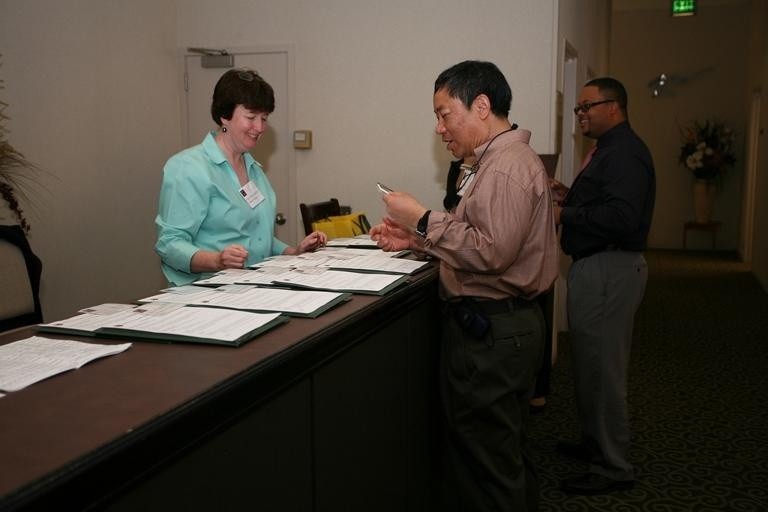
571 243 642 263
444 298 534 314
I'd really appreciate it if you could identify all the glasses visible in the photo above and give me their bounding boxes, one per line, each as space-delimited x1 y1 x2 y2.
573 98 615 116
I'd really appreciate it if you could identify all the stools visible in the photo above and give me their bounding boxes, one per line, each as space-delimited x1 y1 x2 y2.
682 221 723 260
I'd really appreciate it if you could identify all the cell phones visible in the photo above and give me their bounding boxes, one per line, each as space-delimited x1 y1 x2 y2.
376 180 395 195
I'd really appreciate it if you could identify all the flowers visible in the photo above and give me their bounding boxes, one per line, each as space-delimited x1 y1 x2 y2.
673 118 741 197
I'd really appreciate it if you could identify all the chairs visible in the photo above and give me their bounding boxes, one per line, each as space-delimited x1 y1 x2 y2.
0 224 44 335
299 197 341 237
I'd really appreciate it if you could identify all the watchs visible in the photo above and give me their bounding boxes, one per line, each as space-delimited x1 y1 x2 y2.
415 210 430 242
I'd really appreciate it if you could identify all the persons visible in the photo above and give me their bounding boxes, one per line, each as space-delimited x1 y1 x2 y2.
369 61 559 512
154 69 327 287
443 157 551 415
549 77 657 494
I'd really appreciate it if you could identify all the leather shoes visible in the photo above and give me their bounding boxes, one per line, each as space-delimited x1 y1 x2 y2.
556 437 599 462
558 470 636 496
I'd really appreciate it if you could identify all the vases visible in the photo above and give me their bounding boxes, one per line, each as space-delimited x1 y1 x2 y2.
690 176 717 225
